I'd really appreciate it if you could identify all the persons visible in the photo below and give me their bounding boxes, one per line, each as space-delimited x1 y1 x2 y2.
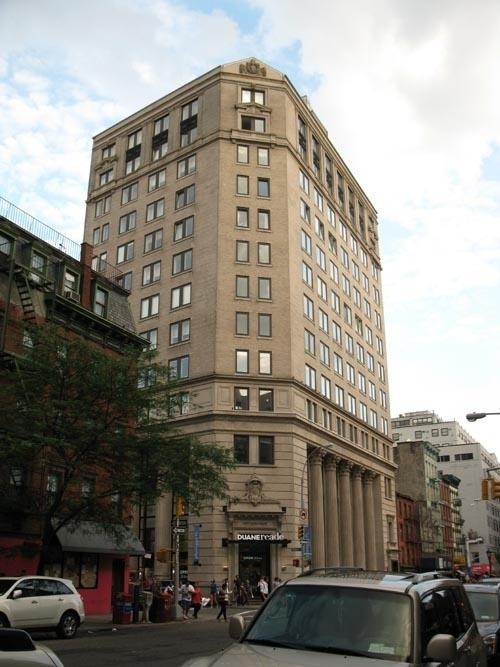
128 572 281 623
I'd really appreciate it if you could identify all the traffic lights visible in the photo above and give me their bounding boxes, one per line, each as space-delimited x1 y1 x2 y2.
298 526 304 539
492 479 500 499
157 548 166 562
177 498 185 517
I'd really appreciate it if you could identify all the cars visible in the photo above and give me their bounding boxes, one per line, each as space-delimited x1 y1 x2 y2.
421 567 500 667
0 575 85 667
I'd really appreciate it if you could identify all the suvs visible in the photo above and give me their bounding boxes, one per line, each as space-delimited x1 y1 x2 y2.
176 566 490 666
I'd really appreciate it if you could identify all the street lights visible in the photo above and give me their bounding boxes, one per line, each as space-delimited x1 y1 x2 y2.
465 537 482 565
429 497 461 569
300 444 333 575
465 412 500 422
486 547 492 576
